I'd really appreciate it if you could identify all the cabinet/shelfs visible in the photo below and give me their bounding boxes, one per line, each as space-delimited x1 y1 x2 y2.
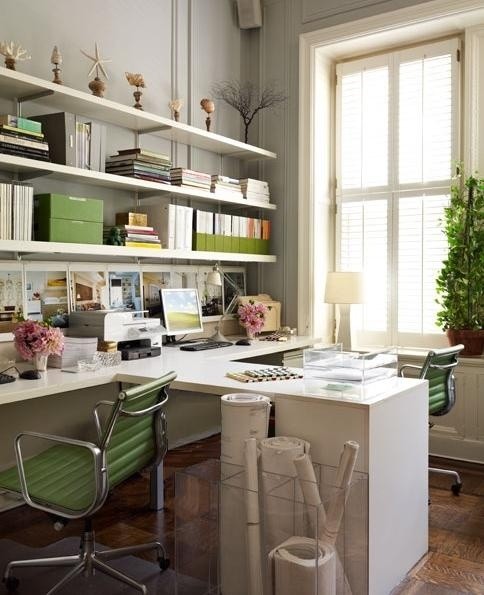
0 62 322 410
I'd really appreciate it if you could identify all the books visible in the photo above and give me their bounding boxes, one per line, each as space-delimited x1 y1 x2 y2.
241 178 270 206
75 120 90 170
0 183 34 241
211 174 243 200
47 336 98 370
195 210 272 257
104 148 171 185
171 167 212 192
150 204 194 252
102 224 162 249
0 114 52 165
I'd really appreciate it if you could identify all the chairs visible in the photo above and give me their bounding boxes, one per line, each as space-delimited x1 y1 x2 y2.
1 371 178 594
395 344 462 504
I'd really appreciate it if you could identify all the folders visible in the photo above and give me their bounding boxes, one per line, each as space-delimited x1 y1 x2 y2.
138 203 194 249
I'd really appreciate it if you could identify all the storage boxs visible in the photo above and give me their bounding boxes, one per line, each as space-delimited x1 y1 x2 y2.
33 193 103 223
115 212 147 227
33 218 103 245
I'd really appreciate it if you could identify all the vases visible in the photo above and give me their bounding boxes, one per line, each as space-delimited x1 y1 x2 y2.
32 353 48 371
246 328 255 341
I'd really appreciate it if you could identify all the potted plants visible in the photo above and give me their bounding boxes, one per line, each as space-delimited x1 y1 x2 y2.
433 157 483 357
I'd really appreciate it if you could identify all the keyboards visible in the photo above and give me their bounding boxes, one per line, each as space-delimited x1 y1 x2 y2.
0 373 16 384
180 341 232 351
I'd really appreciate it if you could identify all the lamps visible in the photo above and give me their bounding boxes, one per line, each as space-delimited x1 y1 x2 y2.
324 271 370 354
205 262 242 342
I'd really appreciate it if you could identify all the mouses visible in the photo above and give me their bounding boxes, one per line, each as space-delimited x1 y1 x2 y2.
20 370 41 380
236 340 249 346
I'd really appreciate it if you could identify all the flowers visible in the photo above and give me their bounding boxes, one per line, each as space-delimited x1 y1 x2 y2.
238 301 269 334
11 320 64 361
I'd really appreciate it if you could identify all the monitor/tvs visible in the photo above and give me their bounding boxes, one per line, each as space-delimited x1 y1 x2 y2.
160 288 204 348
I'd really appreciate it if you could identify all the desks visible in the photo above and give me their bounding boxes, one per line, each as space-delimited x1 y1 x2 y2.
116 359 429 594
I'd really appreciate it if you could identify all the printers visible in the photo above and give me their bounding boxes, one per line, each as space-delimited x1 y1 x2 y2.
69 308 162 362
